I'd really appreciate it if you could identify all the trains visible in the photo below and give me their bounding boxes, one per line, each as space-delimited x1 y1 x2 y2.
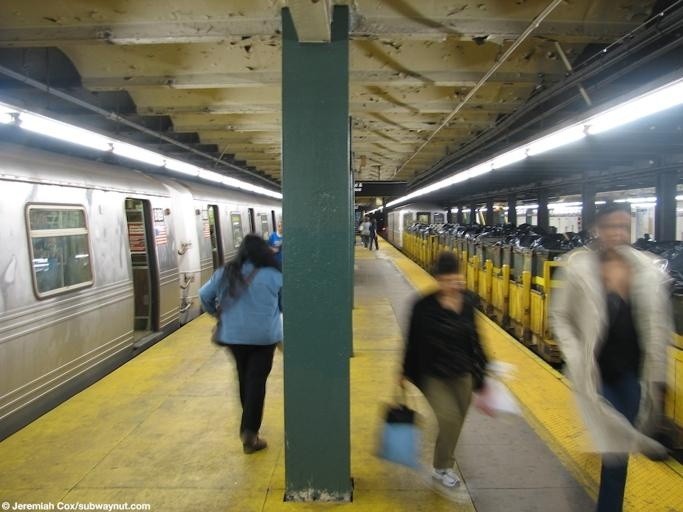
503 203 683 243
0 138 283 441
383 202 683 436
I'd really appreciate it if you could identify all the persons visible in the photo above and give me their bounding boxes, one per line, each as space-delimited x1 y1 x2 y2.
268 221 283 272
357 216 378 251
549 201 671 512
399 251 489 490
197 234 283 454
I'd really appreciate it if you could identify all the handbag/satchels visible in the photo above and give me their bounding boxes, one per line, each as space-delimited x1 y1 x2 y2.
373 386 422 469
210 318 222 346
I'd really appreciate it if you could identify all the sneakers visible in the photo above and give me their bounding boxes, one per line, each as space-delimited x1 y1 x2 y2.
239 427 268 455
432 466 459 487
